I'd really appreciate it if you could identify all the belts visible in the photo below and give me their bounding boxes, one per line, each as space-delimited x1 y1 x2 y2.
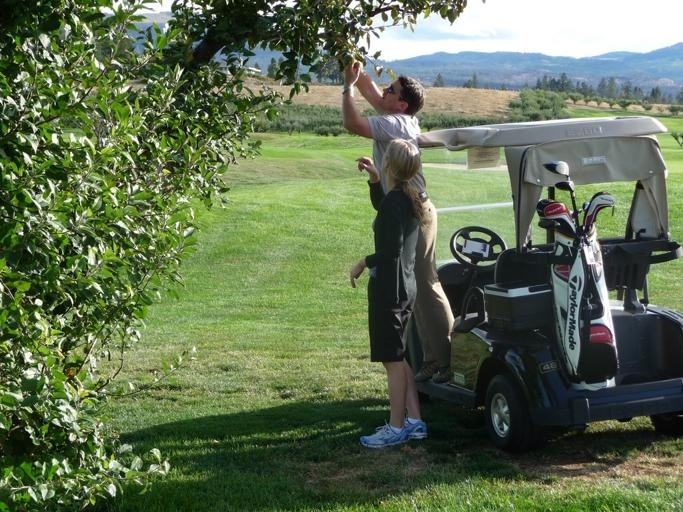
419 191 428 202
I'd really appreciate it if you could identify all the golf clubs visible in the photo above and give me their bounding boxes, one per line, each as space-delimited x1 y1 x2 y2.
537 161 616 236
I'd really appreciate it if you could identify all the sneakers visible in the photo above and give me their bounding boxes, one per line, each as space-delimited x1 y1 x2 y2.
360 423 410 449
376 416 429 440
432 366 451 383
414 362 438 382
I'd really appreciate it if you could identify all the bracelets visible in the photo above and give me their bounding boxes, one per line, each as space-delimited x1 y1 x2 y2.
342 85 352 94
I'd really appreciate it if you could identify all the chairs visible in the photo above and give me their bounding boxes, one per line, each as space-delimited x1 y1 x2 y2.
453 247 550 334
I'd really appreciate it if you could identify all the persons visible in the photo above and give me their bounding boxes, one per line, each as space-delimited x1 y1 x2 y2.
341 54 456 383
350 138 428 449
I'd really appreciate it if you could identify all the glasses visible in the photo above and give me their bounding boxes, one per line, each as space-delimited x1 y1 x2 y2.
387 85 401 100
